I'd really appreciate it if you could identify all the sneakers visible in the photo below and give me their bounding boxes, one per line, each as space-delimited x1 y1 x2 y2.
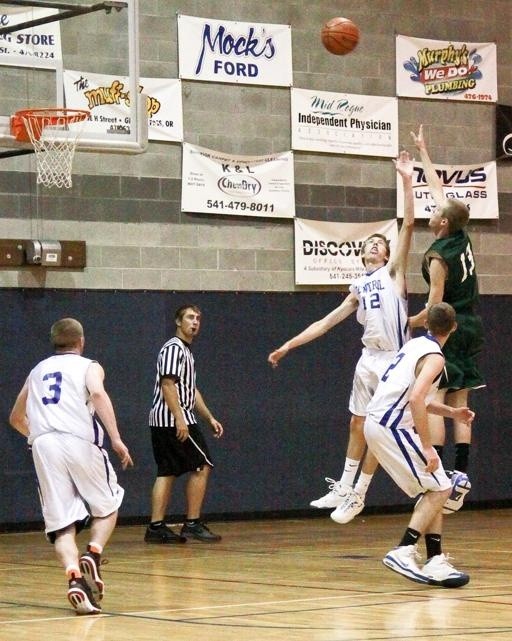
67 551 105 615
181 521 222 542
421 552 469 589
330 493 365 524
441 474 471 517
382 550 430 584
143 522 186 544
310 481 354 509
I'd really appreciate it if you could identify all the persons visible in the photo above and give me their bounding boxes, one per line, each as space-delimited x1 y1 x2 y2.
9 318 134 614
267 150 415 524
408 124 486 514
363 303 475 588
145 304 224 545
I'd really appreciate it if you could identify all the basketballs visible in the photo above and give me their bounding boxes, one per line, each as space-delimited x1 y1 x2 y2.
322 17 360 55
